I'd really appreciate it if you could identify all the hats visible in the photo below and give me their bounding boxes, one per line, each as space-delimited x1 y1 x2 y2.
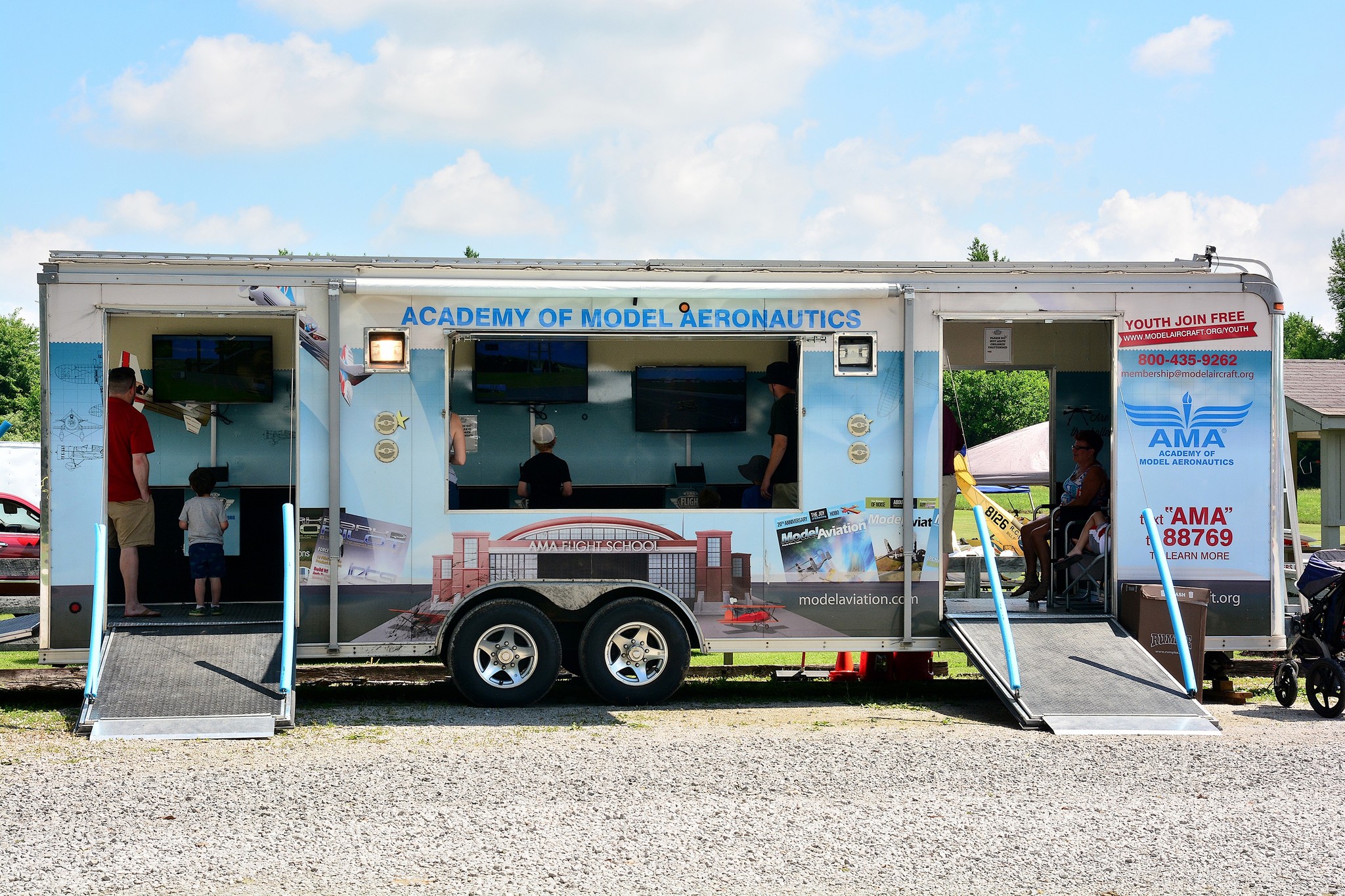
758 361 795 385
532 421 559 445
737 455 770 481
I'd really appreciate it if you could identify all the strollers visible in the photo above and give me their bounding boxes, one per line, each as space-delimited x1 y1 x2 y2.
1273 548 1345 720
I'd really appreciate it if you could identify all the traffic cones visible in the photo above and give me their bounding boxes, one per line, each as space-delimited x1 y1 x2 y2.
828 652 860 682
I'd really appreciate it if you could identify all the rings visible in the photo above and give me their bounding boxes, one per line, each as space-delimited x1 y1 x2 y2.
763 493 764 494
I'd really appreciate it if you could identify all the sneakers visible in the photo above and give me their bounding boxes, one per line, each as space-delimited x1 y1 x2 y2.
188 607 205 616
211 606 223 615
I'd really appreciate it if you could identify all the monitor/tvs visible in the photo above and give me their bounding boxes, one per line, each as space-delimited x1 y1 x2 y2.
472 340 589 403
636 365 748 432
151 334 274 405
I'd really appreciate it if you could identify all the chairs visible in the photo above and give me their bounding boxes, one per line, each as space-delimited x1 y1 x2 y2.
1063 515 1113 614
1029 495 1101 608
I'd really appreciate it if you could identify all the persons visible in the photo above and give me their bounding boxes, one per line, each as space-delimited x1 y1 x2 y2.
1009 430 1109 601
518 423 572 508
179 468 229 616
449 411 466 510
107 367 159 617
943 403 957 611
1053 511 1111 571
738 455 772 509
757 361 798 508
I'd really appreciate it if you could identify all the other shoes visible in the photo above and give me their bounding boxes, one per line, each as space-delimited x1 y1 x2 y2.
943 600 948 613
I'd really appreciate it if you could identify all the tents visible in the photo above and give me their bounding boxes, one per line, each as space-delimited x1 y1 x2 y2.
966 421 1049 487
957 485 1035 511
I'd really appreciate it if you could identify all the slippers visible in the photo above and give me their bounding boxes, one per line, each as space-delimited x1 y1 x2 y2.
125 607 161 618
1054 553 1083 571
1070 537 1091 554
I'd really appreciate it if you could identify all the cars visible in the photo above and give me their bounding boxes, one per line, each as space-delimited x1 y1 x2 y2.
0 492 41 637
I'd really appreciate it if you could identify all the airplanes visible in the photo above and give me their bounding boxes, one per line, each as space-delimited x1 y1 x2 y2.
875 531 926 578
388 608 446 635
716 604 786 631
840 504 861 514
785 551 832 582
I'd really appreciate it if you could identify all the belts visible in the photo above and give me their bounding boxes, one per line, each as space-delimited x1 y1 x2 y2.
942 473 954 476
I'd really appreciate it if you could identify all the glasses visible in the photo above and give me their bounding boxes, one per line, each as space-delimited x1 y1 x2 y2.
1071 445 1092 451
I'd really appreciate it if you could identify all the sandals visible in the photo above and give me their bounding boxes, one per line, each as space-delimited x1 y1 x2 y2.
1010 571 1040 597
1029 573 1056 602
